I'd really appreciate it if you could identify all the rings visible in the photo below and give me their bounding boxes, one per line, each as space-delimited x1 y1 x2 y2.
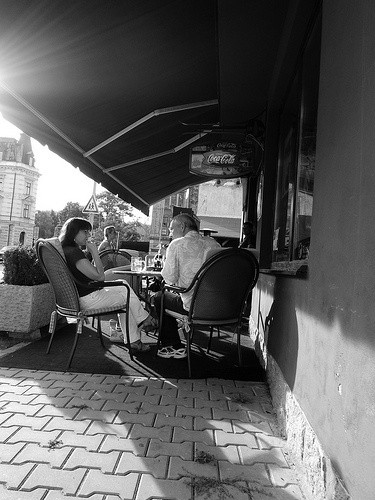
91 242 93 244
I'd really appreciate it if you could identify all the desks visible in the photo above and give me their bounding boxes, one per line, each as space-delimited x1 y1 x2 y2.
113 271 184 345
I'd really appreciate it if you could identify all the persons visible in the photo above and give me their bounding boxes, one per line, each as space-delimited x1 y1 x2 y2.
98 226 120 269
153 213 221 358
59 217 158 355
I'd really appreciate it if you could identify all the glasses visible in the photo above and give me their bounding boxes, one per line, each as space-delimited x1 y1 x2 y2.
83 229 91 234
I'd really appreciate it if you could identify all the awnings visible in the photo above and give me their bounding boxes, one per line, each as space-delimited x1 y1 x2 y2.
0 0 254 217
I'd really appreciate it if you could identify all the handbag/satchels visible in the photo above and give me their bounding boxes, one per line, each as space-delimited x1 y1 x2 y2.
109 318 124 343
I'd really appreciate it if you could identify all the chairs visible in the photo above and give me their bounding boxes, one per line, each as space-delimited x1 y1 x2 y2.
35 238 260 379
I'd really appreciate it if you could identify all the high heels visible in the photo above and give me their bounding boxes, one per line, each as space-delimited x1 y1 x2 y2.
141 317 169 335
129 342 152 361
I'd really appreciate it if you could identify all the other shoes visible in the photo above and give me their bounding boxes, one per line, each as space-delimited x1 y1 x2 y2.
157 346 188 359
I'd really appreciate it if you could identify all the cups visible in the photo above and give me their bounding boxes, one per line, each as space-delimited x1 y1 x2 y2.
131 257 142 272
